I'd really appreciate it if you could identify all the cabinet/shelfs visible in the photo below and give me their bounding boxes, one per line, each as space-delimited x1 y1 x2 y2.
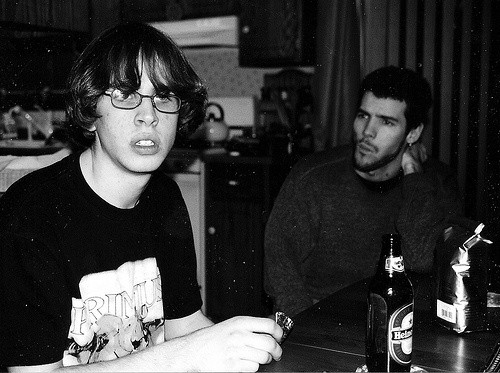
237 0 317 67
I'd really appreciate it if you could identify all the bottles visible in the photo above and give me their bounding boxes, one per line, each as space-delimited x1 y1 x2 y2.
364 231 415 373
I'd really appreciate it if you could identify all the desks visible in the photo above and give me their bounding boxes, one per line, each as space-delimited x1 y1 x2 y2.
255 263 500 373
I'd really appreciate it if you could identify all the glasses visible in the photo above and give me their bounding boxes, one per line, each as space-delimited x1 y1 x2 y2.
103 87 185 113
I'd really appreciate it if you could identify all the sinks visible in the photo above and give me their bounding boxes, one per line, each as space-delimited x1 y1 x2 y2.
11 125 66 143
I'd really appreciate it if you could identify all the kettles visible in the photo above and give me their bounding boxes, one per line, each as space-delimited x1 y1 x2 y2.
202 104 229 143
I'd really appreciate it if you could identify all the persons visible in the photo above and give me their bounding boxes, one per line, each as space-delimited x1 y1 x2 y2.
261 64 481 322
0 23 283 373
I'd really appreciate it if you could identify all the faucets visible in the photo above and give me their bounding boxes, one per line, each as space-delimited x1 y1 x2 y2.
7 106 21 138
